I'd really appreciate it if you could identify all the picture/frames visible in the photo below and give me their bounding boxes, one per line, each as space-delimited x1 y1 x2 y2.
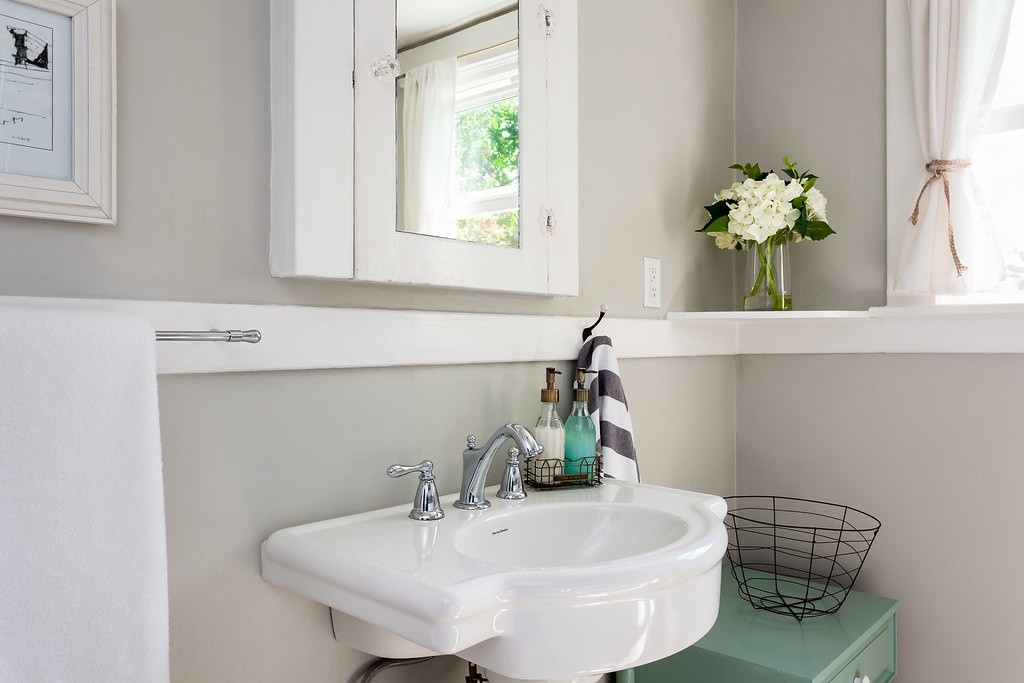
0 1 117 225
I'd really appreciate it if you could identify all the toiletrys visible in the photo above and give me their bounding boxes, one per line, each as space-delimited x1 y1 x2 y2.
528 367 564 483
564 367 599 477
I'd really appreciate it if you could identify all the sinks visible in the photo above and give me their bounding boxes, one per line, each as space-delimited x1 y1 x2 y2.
262 478 727 683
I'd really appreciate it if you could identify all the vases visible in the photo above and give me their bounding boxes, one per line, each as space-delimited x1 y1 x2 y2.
743 232 792 311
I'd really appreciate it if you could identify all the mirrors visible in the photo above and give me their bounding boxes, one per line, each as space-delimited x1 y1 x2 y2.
395 1 521 249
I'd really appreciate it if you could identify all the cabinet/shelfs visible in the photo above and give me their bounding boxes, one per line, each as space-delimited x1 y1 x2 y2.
616 565 899 683
269 0 580 298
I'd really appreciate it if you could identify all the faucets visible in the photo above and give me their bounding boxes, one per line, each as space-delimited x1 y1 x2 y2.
454 424 543 507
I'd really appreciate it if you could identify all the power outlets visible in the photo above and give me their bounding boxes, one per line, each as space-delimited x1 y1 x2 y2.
644 257 661 309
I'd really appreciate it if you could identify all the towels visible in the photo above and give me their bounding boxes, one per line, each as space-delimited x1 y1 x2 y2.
576 335 640 482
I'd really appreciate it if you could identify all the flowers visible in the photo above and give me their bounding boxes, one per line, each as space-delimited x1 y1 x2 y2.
694 154 838 249
